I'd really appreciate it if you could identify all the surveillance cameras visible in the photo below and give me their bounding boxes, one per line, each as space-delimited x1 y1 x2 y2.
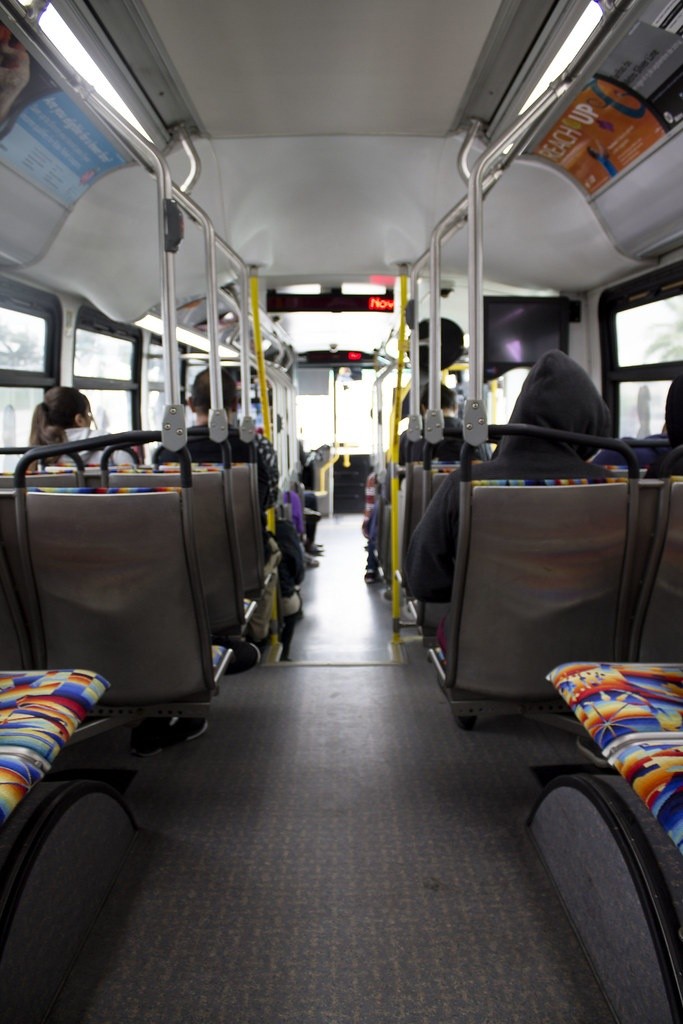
439 279 456 298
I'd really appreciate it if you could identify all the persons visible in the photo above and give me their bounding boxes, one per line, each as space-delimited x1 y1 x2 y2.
25 370 323 756
364 383 492 602
403 350 683 731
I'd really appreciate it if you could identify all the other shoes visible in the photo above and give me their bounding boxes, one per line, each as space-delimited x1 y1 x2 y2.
365 573 375 582
306 545 323 553
216 637 262 674
133 718 207 757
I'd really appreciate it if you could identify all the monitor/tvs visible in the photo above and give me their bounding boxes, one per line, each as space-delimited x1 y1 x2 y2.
484 295 570 367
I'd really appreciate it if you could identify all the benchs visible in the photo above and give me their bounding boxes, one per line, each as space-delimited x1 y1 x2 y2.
364 373 683 1024
0 429 334 965
